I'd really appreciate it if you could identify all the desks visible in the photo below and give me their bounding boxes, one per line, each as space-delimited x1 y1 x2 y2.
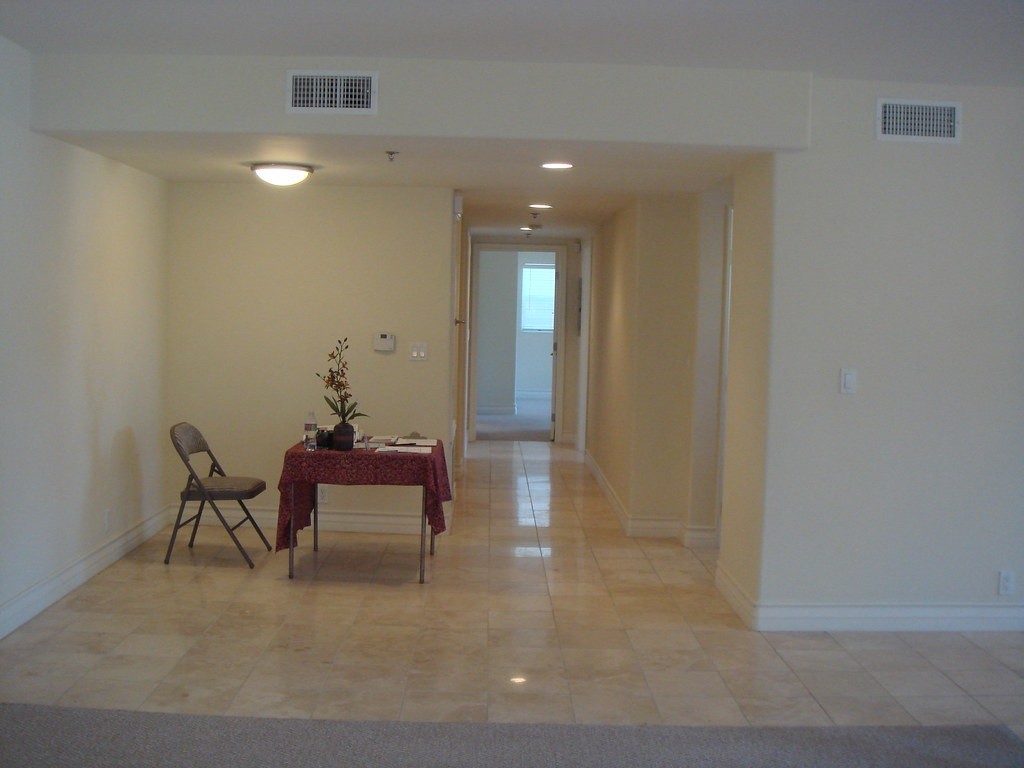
276 435 453 584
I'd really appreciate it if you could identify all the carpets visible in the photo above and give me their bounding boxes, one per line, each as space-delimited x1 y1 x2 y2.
1 704 1024 768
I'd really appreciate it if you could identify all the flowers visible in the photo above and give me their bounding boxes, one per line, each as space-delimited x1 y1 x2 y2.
316 337 370 423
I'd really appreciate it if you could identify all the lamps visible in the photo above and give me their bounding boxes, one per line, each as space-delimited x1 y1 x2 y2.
251 164 313 187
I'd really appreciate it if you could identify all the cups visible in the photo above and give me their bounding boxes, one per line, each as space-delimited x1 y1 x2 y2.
316 430 334 449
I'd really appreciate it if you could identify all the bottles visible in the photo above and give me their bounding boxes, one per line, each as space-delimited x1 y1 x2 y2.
304 411 317 451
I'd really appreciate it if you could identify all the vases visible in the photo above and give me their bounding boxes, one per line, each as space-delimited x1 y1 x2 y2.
334 422 354 450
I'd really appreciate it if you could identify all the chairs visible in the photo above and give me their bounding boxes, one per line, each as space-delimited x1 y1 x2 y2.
165 422 271 569
